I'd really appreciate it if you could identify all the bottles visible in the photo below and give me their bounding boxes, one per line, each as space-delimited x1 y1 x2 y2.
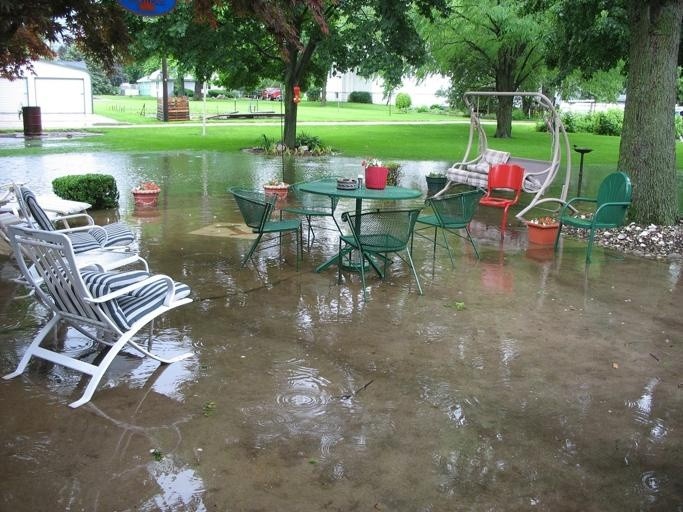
357 174 364 190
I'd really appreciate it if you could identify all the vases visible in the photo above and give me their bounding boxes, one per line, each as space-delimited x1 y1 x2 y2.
364 167 388 190
527 221 558 245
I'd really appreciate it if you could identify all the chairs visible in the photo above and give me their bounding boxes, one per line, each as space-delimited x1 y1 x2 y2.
553 170 633 266
467 163 525 241
227 175 485 304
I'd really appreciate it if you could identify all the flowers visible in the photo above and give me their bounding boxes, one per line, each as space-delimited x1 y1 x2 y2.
528 214 556 226
360 156 380 170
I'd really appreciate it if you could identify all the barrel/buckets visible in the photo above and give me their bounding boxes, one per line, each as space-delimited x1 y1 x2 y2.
366 167 389 188
426 177 447 194
23 106 41 134
